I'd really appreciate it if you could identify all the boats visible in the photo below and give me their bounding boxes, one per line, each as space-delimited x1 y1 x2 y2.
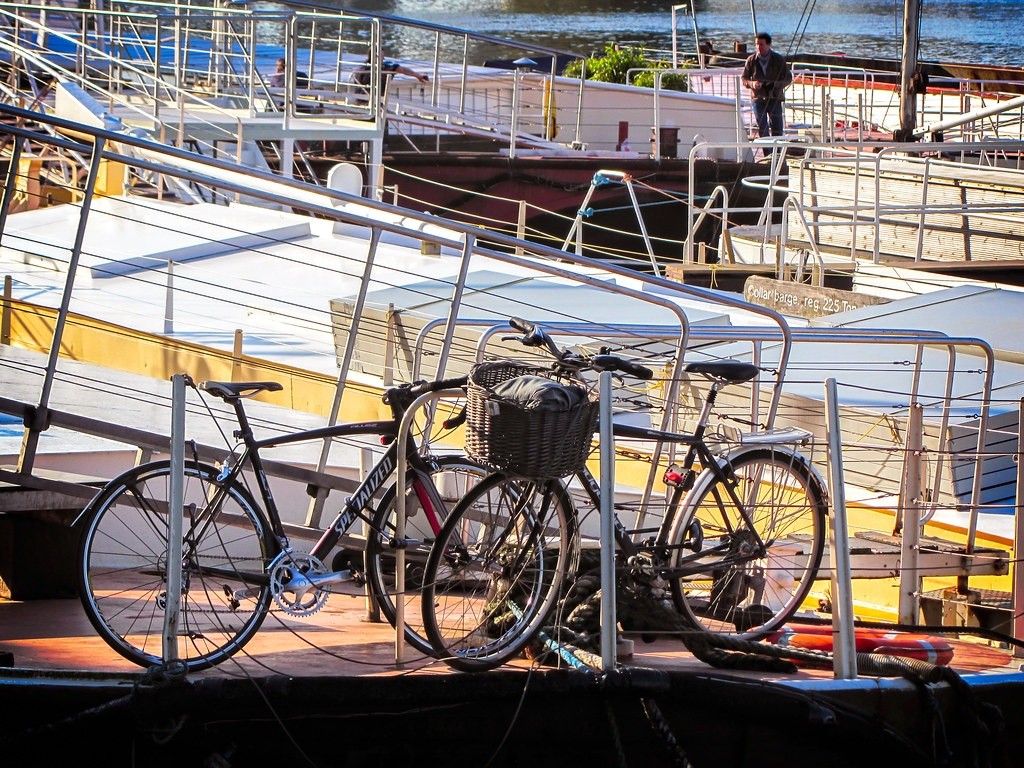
0 0 1023 642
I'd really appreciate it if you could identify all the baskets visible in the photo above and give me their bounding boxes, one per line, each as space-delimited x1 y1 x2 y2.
466 361 597 481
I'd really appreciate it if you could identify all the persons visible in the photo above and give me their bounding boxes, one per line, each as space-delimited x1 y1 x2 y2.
741 32 795 159
346 45 430 109
268 56 288 86
704 38 721 57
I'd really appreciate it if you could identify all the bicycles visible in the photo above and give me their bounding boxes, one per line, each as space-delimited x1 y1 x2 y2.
68 377 548 679
415 315 831 678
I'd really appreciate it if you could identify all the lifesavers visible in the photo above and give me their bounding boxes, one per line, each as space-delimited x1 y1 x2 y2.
766 626 954 665
